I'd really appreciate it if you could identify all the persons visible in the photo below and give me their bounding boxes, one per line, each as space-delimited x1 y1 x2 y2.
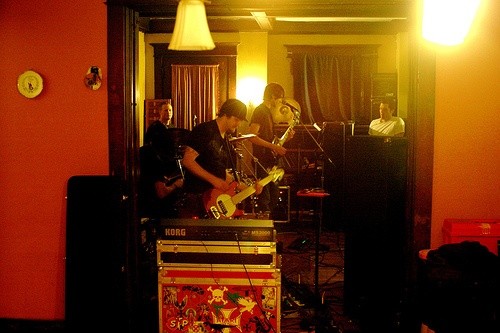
369 99 405 137
248 83 295 217
181 99 262 218
144 102 201 301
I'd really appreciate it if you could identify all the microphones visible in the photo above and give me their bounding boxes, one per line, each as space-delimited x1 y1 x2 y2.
282 100 299 113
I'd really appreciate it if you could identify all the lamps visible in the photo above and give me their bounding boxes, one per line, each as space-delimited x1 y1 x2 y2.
167 0 216 51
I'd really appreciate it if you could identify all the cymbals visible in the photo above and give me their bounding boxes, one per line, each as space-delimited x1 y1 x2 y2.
267 98 303 124
226 135 259 141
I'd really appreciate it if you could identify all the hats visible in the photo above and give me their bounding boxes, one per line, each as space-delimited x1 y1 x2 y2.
220 98 248 121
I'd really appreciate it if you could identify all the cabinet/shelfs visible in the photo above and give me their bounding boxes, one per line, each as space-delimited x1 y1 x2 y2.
274 122 325 215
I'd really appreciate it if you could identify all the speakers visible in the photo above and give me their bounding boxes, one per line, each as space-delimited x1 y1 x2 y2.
344 134 412 322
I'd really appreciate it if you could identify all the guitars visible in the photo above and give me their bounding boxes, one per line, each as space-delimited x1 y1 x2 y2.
251 110 300 176
202 170 284 219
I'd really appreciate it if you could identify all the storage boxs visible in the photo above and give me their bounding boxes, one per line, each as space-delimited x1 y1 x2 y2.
442 218 500 255
